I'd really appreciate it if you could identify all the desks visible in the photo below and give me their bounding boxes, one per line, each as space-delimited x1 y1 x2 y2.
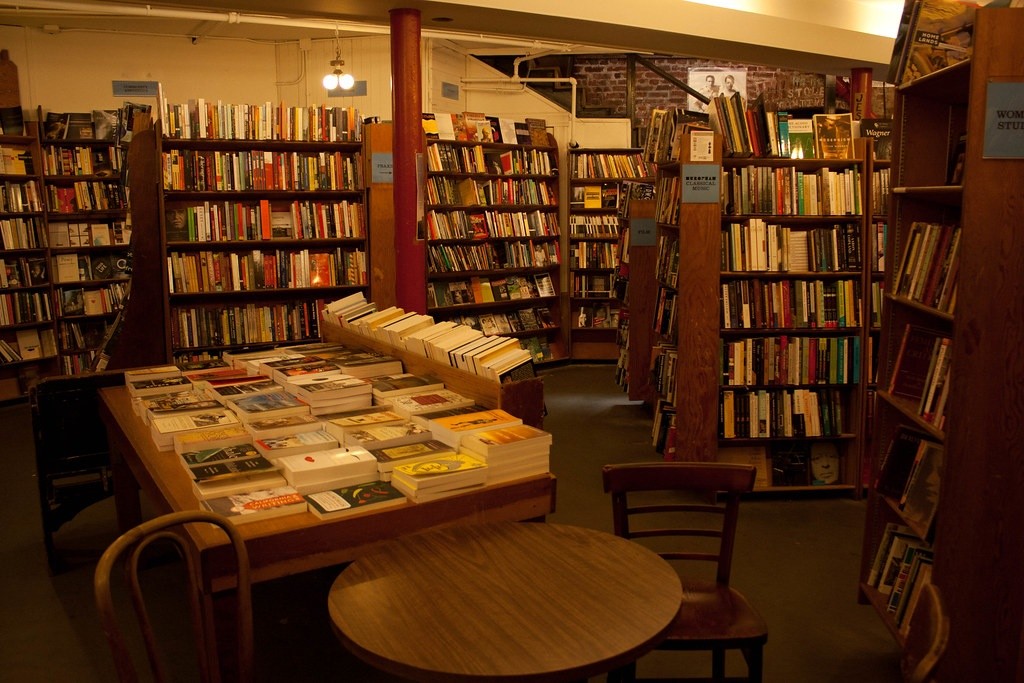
325 522 683 683
97 386 559 599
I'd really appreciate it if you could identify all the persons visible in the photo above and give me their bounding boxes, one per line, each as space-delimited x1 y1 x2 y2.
694 74 738 111
811 442 839 484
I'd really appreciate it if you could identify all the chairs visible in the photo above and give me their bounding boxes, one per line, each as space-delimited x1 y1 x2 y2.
602 462 768 683
94 511 255 683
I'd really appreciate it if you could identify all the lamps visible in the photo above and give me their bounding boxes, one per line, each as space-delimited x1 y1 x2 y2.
323 22 354 90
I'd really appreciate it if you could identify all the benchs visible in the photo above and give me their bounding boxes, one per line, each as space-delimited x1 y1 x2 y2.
28 357 224 577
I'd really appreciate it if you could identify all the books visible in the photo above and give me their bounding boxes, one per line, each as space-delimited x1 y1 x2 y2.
422 111 557 205
885 1 1024 318
167 247 366 292
569 152 653 394
172 299 326 347
887 324 955 431
722 217 887 486
125 341 553 525
45 102 153 375
162 150 363 192
319 291 550 420
865 521 934 639
156 82 362 142
640 104 713 453
426 210 562 362
707 96 898 216
166 198 365 241
873 423 942 549
0 106 58 363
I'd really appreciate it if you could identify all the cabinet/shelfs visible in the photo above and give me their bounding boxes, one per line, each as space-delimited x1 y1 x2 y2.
1 7 1024 683
